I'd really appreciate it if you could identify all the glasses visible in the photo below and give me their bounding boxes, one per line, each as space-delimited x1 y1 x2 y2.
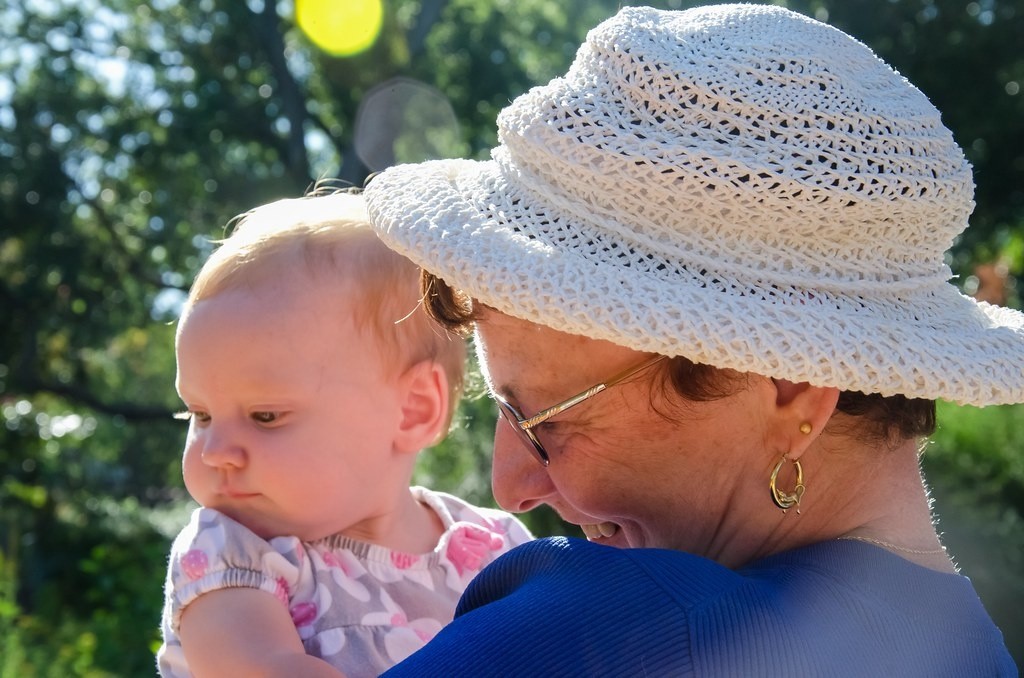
495 353 666 468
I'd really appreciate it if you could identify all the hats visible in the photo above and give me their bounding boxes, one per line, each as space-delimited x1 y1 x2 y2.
363 4 1024 408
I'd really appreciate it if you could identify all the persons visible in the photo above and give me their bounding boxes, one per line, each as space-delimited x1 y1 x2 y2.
155 187 539 678
366 0 1024 678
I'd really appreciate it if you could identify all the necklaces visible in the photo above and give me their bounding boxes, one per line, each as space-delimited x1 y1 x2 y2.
839 535 946 554
